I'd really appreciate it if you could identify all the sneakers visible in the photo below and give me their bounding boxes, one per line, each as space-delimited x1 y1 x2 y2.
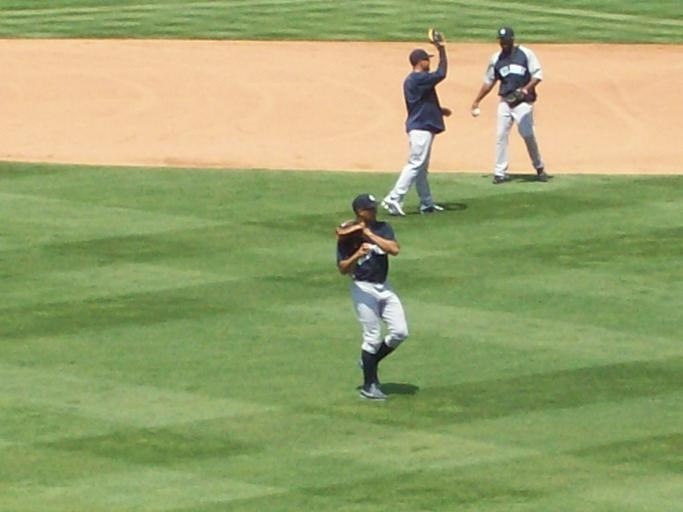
538 168 548 182
492 175 510 183
381 197 405 215
420 205 443 214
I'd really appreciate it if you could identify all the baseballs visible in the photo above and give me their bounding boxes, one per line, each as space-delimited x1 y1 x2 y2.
364 244 373 255
473 109 480 117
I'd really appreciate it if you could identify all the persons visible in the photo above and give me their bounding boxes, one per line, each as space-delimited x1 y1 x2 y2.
377 26 454 217
470 25 554 184
335 193 410 402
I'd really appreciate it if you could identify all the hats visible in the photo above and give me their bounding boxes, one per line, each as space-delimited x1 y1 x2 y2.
410 49 434 64
496 27 514 40
353 193 380 211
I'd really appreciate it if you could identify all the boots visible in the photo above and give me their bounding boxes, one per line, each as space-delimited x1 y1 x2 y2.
359 339 394 388
361 349 388 401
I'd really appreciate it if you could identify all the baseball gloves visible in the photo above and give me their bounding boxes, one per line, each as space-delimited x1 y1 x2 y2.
336 218 367 240
428 29 446 49
506 86 530 107
